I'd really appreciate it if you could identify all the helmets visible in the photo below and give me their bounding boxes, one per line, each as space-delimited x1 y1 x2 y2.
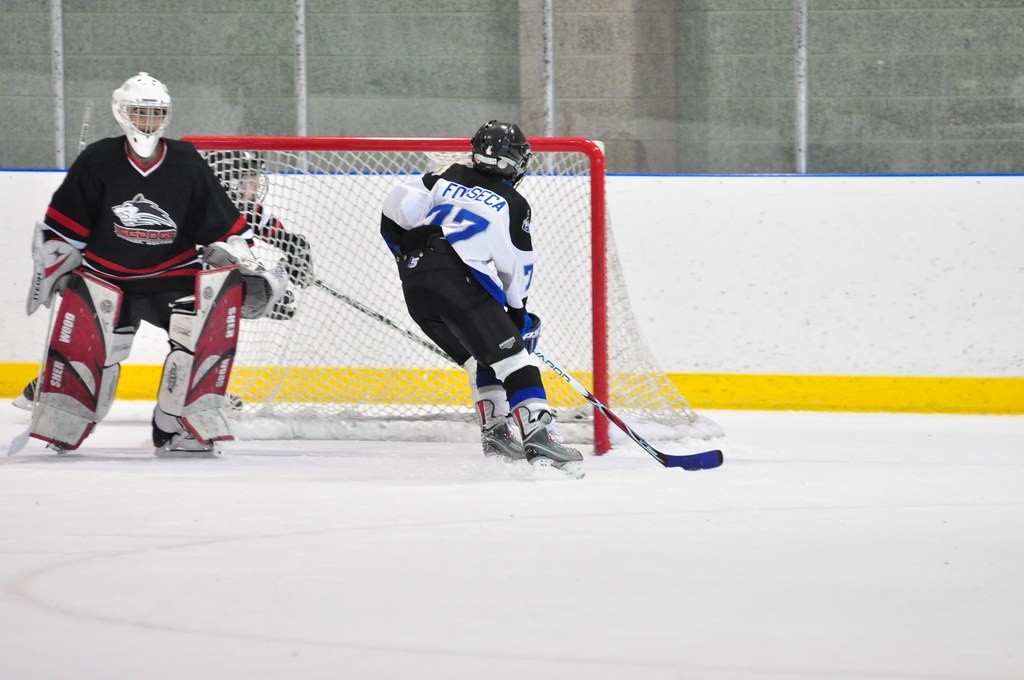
470 119 533 189
204 149 270 212
110 71 172 159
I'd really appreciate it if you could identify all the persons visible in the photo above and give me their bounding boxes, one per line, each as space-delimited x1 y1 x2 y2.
378 122 585 463
22 69 320 455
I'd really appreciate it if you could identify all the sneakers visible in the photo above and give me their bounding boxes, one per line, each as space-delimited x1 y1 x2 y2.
511 404 587 481
481 420 524 469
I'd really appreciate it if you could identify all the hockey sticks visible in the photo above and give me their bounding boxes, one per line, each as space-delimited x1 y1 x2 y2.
5 122 90 458
310 274 594 423
533 344 725 471
